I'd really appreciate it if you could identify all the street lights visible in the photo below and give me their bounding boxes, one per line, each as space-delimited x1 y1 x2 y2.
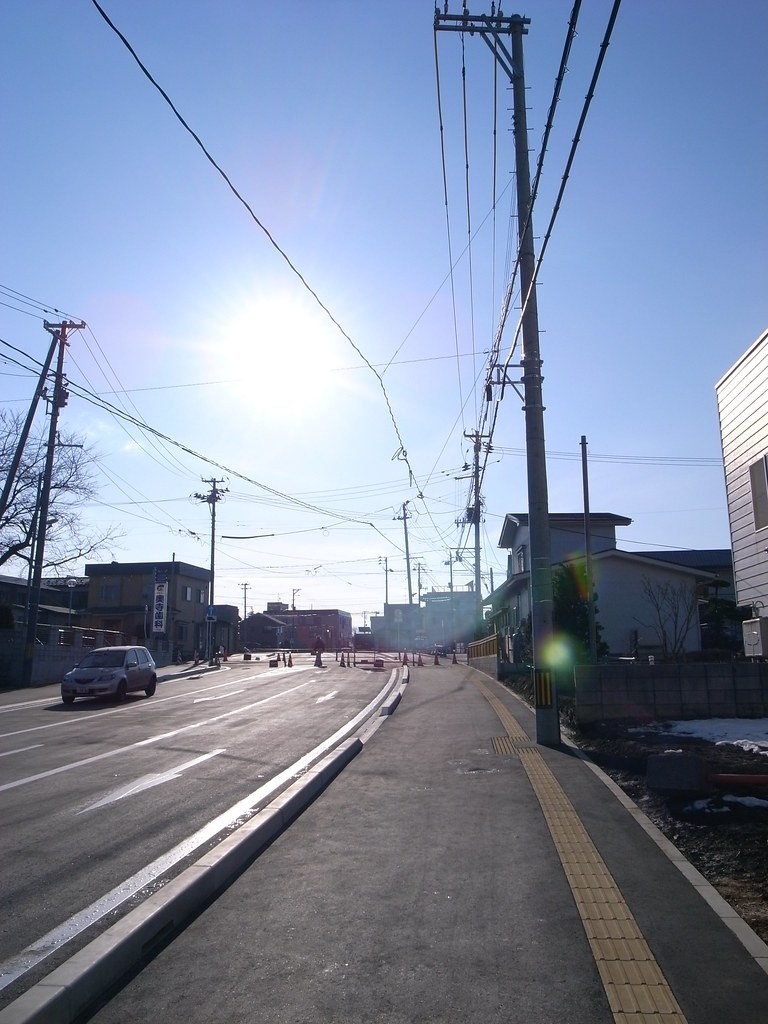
66 578 77 624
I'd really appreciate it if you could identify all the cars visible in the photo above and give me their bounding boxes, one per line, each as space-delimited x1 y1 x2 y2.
61 646 157 704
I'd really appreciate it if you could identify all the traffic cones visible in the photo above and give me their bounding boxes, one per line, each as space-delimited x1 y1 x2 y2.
208 650 228 666
276 650 351 668
402 648 458 667
174 648 185 665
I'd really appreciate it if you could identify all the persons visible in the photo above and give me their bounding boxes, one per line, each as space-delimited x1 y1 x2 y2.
315 636 324 653
